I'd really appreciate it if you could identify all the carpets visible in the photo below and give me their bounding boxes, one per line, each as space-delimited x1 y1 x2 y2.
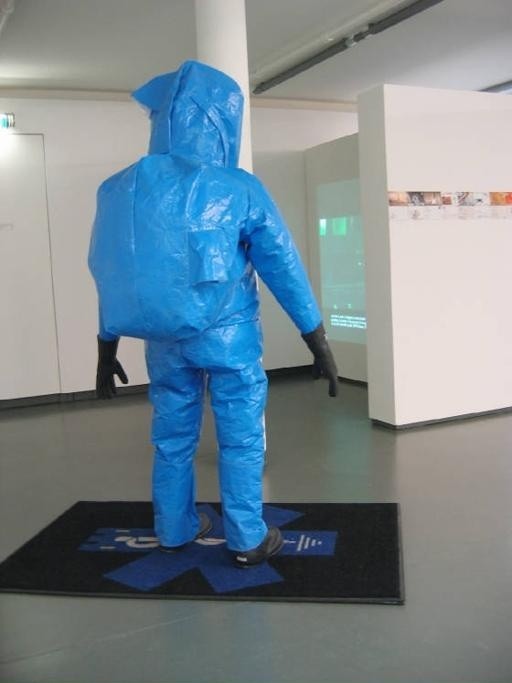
0 499 406 606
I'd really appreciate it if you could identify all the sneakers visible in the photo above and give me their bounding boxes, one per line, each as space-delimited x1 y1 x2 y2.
235 526 285 568
158 513 214 555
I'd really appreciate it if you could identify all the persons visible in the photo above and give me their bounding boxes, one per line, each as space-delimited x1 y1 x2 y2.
85 56 341 570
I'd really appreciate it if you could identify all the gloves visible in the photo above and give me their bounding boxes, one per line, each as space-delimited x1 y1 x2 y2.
94 336 128 401
301 322 338 398
